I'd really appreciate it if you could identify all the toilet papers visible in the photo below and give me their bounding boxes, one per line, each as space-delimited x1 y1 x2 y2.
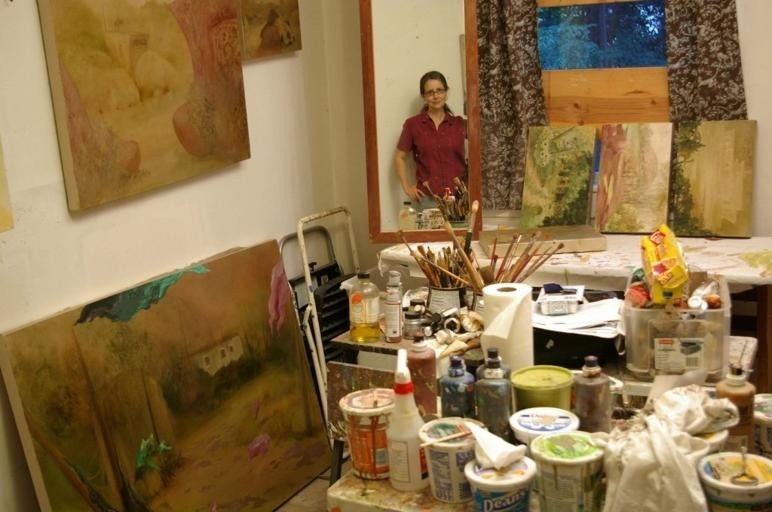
479 282 534 369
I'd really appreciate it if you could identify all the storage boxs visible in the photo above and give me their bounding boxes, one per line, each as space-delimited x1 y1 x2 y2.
624 268 732 383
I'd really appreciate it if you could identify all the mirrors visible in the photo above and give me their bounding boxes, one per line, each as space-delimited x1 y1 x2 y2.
358 0 483 245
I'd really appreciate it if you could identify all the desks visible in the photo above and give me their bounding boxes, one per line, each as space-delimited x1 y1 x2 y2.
376 216 771 394
325 301 758 488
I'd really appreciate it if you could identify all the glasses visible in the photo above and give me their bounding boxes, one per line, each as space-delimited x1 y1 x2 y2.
424 88 447 96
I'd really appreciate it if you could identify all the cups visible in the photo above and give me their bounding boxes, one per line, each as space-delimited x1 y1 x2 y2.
754 394 772 459
339 389 395 479
510 408 580 456
531 430 604 512
420 417 487 502
699 452 772 512
465 456 537 512
511 365 573 414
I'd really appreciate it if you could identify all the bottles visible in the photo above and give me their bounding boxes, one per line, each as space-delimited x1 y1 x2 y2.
385 369 431 491
573 355 610 432
476 349 511 440
349 270 376 344
385 272 406 344
714 362 757 452
439 353 475 420
398 332 438 421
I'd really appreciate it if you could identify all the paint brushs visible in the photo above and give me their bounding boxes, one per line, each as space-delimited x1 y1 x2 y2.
442 220 565 295
421 175 470 222
396 199 480 288
420 423 496 453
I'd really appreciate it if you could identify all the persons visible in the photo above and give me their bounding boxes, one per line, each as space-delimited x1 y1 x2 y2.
394 72 468 221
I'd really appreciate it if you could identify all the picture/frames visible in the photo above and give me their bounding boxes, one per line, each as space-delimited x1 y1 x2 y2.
0 240 336 508
239 1 303 63
668 120 758 238
35 1 253 214
520 124 596 228
593 121 672 235
